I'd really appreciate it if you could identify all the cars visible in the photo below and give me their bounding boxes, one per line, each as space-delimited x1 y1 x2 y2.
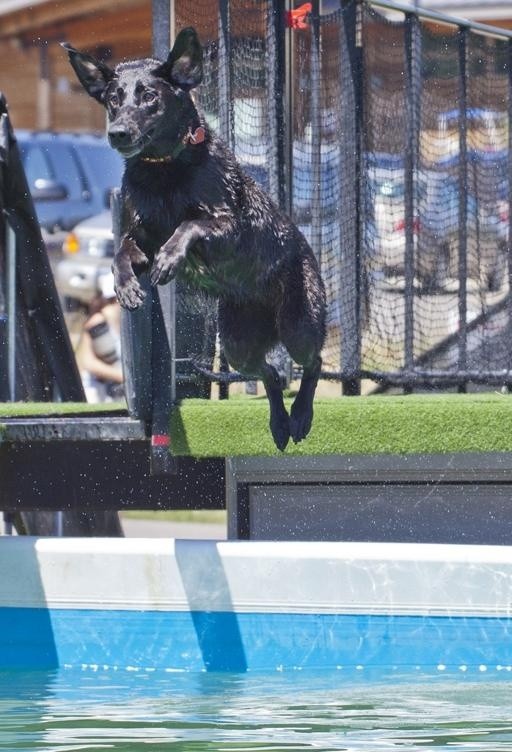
11 109 510 333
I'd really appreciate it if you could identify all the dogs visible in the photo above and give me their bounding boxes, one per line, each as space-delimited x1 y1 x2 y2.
62 25 328 455
